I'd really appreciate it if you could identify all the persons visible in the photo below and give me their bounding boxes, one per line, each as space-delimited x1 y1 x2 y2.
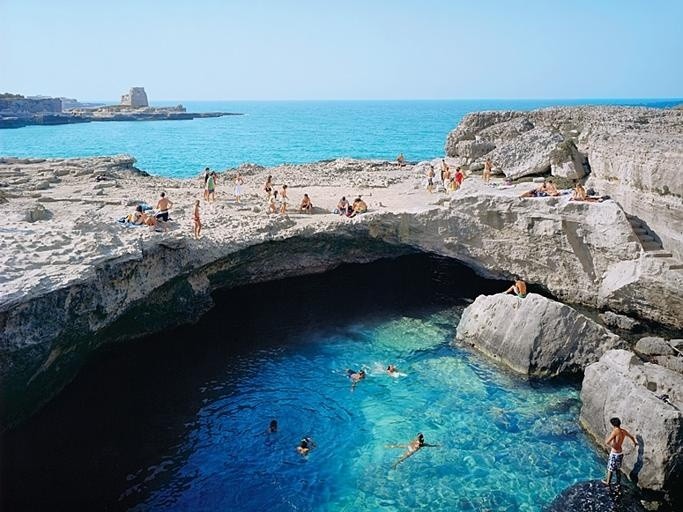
396 152 404 166
422 155 591 202
344 367 364 392
386 432 440 471
295 439 316 455
599 417 639 486
386 365 397 375
153 192 174 222
191 200 201 238
131 205 147 226
268 419 278 432
502 276 526 298
197 163 373 218
